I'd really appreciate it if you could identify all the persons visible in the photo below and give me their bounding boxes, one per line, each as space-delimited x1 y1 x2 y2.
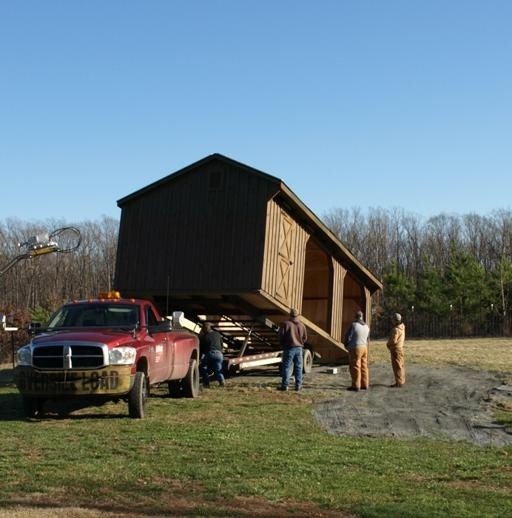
197 321 226 389
344 309 371 391
386 312 408 388
277 307 307 391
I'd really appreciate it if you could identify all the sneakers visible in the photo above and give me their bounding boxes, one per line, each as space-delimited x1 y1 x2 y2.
296 385 303 390
277 385 287 391
347 385 360 391
391 381 400 387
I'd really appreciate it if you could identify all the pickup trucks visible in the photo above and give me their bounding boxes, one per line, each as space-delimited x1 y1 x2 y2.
13 290 201 419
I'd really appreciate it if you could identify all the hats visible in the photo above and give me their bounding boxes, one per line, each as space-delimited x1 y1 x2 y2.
393 312 401 321
291 308 299 317
